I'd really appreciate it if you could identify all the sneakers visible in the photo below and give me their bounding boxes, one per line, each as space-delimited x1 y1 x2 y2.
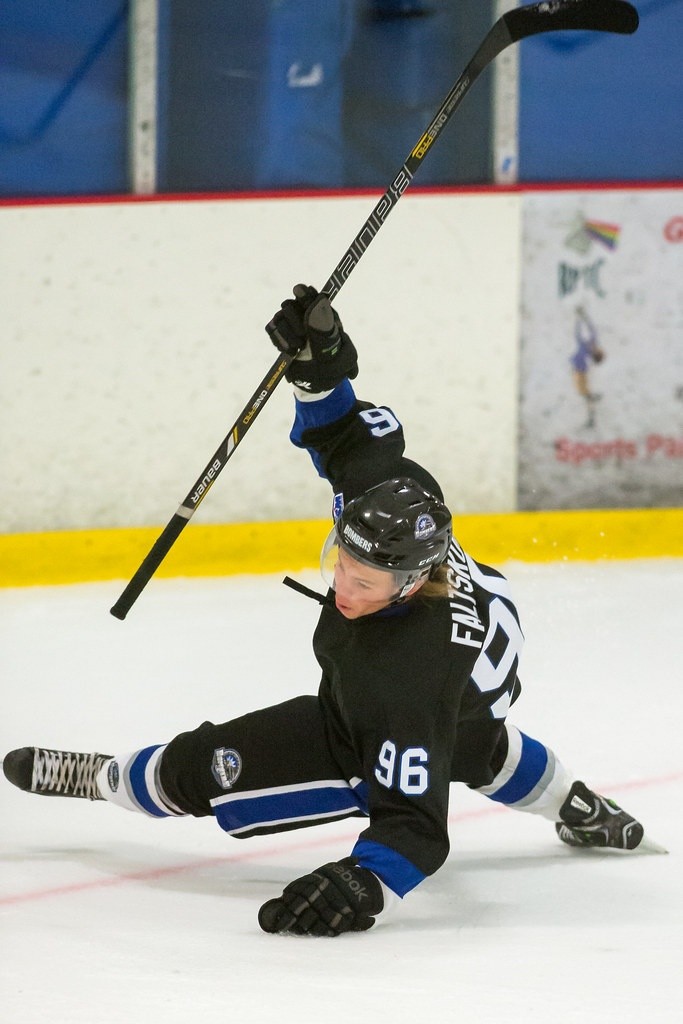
2 746 114 800
555 780 644 851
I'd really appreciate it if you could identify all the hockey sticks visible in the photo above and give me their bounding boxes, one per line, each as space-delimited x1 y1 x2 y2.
109 0 641 621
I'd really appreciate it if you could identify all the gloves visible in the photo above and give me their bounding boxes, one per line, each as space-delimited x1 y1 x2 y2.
272 858 383 937
266 284 358 394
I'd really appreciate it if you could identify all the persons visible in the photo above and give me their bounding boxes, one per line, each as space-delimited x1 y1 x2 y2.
2 283 670 936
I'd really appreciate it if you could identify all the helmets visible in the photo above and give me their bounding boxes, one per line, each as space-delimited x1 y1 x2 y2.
335 478 452 572
